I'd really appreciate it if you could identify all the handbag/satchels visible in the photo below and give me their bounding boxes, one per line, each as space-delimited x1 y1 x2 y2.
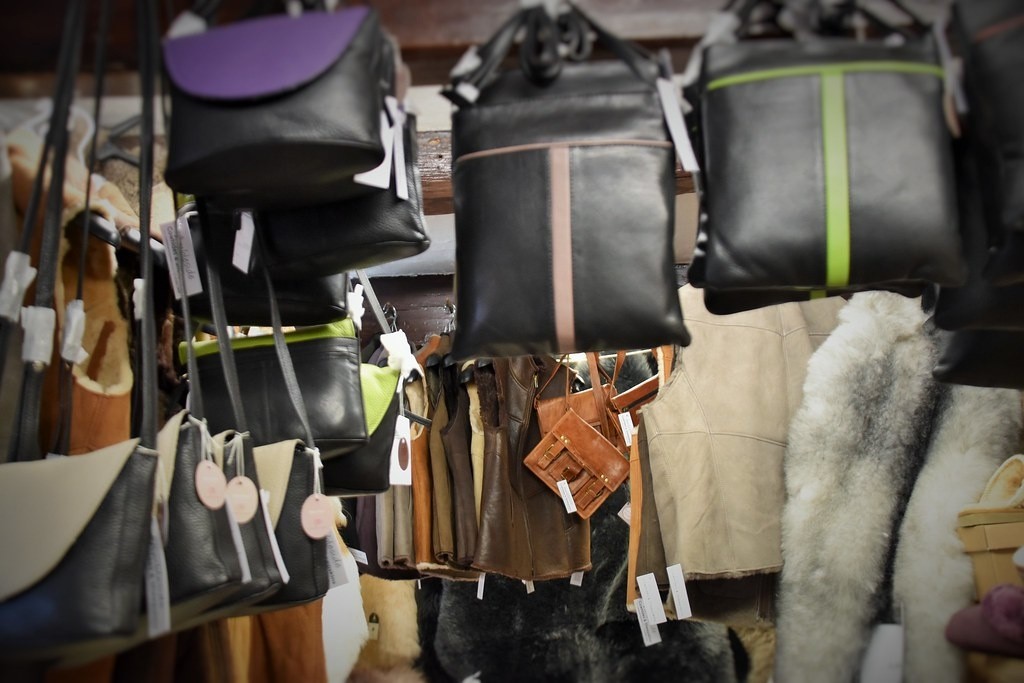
0 0 433 673
608 345 662 426
532 350 631 454
523 350 631 520
679 0 1024 385
441 3 691 361
